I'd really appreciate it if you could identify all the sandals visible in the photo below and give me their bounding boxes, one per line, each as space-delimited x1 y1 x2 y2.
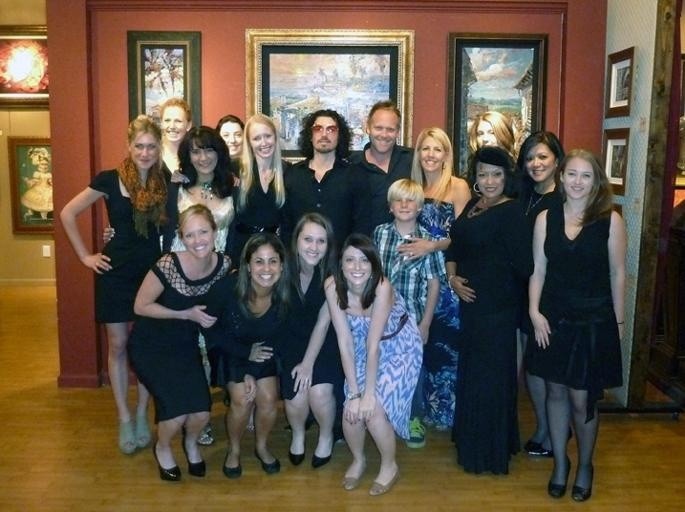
572 466 594 502
548 458 571 498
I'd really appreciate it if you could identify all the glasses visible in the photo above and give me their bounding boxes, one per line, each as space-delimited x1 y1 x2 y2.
311 125 339 135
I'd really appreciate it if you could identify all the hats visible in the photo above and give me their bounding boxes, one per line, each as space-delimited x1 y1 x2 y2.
477 147 509 167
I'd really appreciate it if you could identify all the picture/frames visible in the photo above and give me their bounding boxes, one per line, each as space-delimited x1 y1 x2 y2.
604 47 634 118
447 31 548 189
127 30 201 133
674 53 685 189
0 25 49 107
7 135 53 234
244 28 415 166
602 127 628 196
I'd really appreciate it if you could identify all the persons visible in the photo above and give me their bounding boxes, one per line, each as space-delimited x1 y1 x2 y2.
61 93 627 505
21 154 54 220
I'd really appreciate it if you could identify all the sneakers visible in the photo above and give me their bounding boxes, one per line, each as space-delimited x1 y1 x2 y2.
404 416 427 448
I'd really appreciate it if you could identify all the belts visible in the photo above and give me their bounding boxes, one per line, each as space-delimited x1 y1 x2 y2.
233 218 278 235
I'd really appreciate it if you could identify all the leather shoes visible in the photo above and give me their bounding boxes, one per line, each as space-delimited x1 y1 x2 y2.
524 426 573 456
119 414 400 495
